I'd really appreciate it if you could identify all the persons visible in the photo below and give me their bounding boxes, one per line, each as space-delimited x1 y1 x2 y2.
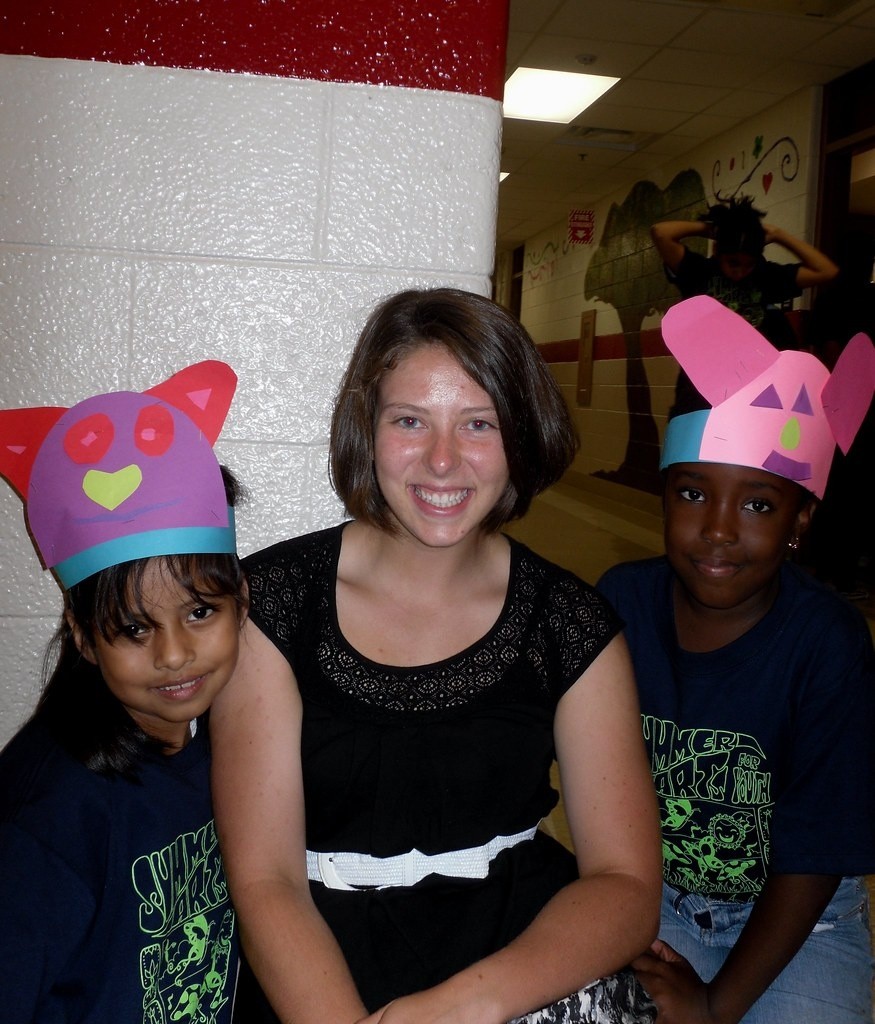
209 286 665 1024
1 359 241 1023
577 193 875 1024
